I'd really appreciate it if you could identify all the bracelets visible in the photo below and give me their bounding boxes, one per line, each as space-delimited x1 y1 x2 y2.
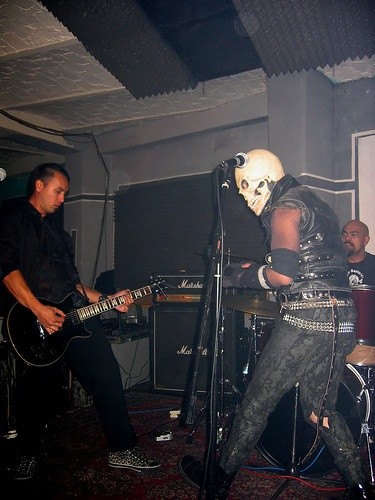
98 293 107 302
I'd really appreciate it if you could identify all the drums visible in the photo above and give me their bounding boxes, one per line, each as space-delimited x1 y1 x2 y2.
343 283 375 367
256 356 375 475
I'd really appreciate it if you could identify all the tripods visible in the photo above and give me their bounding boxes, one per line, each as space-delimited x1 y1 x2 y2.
249 380 346 500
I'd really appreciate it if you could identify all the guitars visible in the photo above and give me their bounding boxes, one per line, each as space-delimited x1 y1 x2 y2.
5 275 170 368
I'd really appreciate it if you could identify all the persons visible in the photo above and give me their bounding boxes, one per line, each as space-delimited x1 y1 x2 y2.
0 162 161 482
180 149 375 500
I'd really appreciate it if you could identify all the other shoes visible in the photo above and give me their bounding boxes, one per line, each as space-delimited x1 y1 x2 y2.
106 447 163 473
13 455 40 482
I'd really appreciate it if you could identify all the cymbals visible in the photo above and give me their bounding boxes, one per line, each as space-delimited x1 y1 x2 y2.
213 293 282 318
195 249 248 258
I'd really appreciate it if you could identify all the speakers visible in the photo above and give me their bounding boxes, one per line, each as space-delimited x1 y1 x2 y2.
102 322 149 389
148 304 244 399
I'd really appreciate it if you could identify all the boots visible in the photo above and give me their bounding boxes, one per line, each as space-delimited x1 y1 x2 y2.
327 473 375 500
179 454 239 500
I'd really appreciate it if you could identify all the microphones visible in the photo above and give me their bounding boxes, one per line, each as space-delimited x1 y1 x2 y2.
218 152 248 169
0 168 7 181
